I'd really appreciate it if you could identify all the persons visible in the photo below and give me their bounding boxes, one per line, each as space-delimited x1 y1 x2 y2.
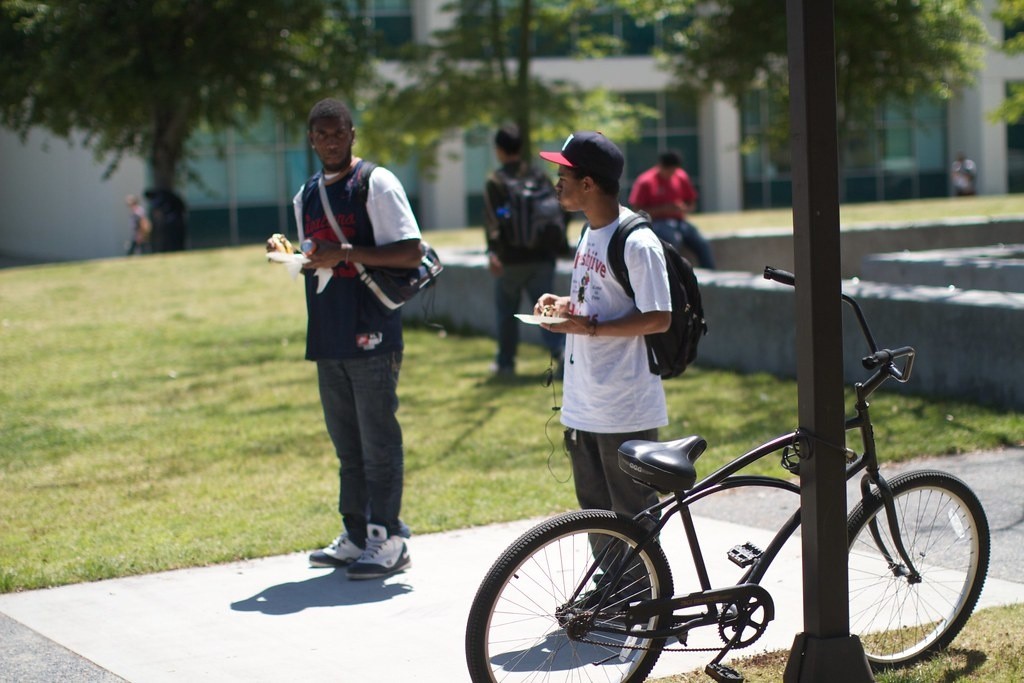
540 128 672 629
950 152 977 197
267 99 425 580
481 122 566 376
629 151 717 270
126 186 185 258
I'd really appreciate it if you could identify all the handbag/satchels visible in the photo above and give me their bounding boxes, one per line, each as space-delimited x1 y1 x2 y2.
358 162 444 310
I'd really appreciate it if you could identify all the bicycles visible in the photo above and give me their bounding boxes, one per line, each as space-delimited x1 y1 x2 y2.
463 263 989 683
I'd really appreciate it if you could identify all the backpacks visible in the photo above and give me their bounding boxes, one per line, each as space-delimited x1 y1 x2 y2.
494 167 569 262
581 207 708 380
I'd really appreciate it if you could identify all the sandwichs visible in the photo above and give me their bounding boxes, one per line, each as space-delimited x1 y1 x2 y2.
272 233 293 254
542 304 588 320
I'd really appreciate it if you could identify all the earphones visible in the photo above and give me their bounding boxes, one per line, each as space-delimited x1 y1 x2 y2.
570 354 574 365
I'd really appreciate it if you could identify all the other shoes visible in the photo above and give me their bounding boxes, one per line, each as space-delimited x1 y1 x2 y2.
556 593 630 614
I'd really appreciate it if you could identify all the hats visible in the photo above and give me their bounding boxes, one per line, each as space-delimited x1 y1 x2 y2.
539 129 625 187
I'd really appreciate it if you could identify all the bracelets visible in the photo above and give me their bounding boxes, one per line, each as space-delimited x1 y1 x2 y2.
590 314 599 337
346 242 349 263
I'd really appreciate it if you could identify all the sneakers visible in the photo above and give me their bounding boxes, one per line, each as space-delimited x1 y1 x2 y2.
346 523 411 579
309 529 364 567
